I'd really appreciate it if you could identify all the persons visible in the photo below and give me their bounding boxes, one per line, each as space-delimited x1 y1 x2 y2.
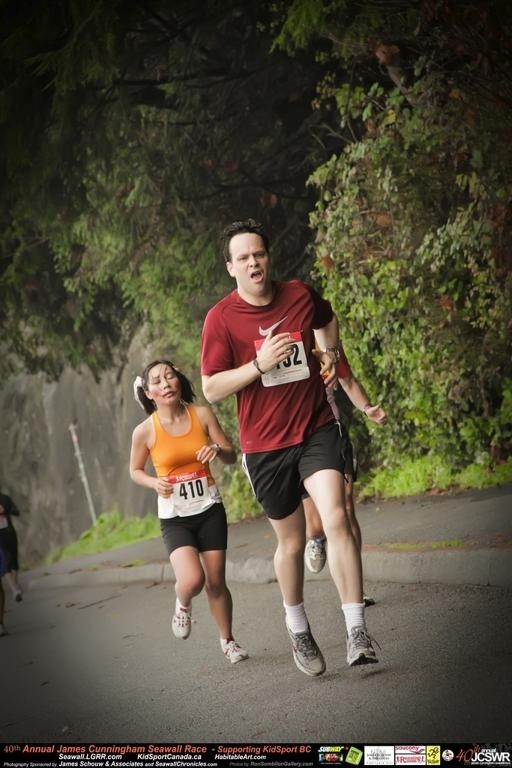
304 334 389 607
128 359 249 665
1 578 10 637
0 490 24 602
199 220 382 677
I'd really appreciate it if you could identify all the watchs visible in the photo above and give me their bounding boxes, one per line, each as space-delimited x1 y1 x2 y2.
322 347 341 364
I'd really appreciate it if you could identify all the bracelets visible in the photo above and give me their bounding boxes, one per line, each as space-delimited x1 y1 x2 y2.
214 442 222 455
253 358 265 375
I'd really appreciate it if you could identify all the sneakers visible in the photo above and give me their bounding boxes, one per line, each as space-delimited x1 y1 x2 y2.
171 597 191 640
347 626 378 666
220 638 249 664
304 534 326 573
285 615 326 676
